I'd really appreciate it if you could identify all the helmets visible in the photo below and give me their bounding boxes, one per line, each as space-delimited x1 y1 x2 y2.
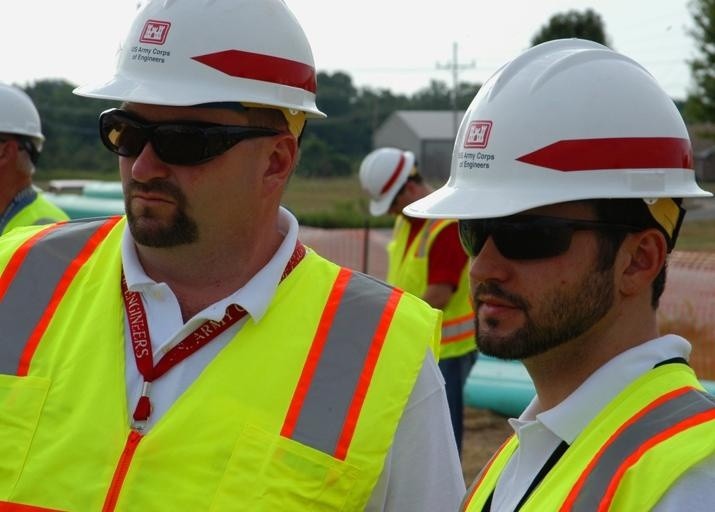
402 36 714 220
358 147 415 217
71 0 330 119
0 85 45 153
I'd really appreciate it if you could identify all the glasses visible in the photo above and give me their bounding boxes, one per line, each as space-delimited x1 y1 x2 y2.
457 216 654 261
97 107 282 166
390 191 401 210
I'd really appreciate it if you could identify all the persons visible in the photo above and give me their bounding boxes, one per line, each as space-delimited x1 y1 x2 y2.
0 82 69 236
0 0 469 512
402 38 715 512
359 147 478 448
